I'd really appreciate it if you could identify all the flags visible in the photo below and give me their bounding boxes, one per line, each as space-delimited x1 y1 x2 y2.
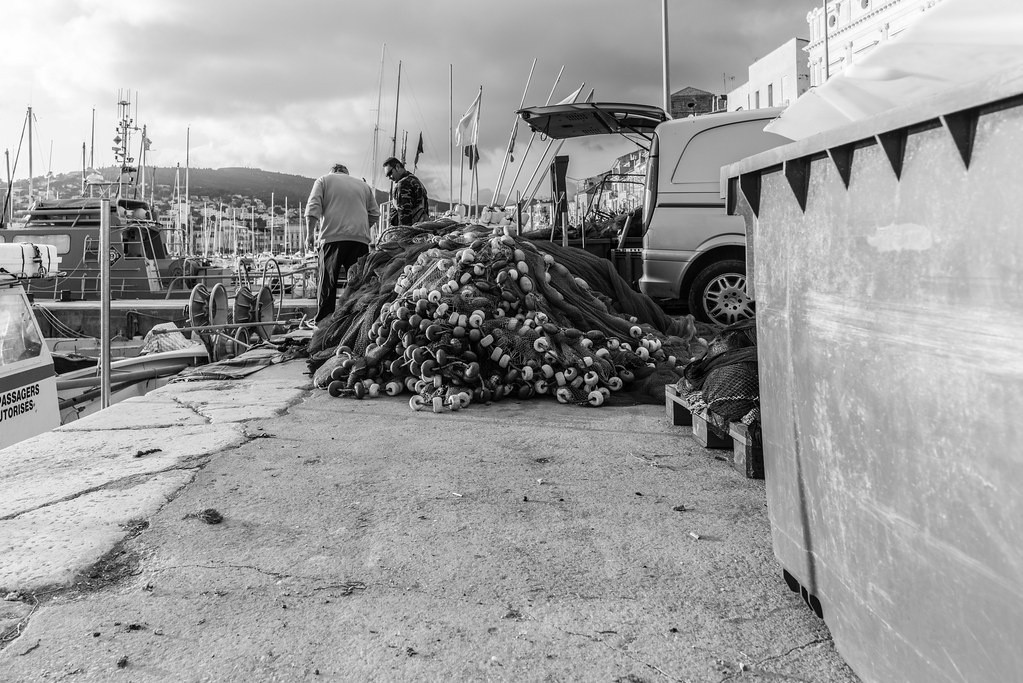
414 133 423 167
455 91 481 146
465 145 480 169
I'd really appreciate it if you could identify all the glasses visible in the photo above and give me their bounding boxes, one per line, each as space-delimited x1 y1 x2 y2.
386 164 396 177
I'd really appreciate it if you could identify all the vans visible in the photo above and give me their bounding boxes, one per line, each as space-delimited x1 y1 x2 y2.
517 99 788 327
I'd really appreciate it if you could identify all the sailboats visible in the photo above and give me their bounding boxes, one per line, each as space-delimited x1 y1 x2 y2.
0 86 319 449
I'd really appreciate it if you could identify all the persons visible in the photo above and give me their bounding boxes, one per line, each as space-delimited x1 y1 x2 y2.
384 158 429 228
305 163 381 326
